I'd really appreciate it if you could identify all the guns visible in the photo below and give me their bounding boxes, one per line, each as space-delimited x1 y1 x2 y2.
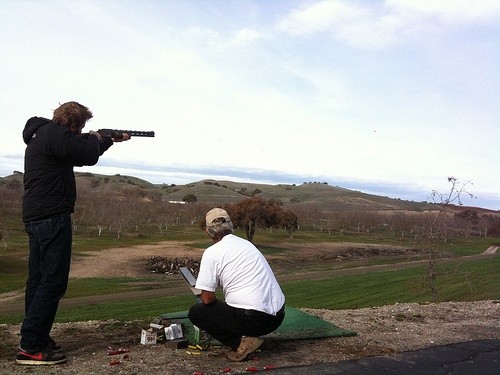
89 128 155 139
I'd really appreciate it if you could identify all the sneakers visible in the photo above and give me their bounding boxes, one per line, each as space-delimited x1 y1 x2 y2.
15 347 67 366
46 341 62 352
226 335 263 361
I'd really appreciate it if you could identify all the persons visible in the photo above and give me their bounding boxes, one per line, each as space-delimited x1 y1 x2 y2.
13 101 131 365
187 208 285 361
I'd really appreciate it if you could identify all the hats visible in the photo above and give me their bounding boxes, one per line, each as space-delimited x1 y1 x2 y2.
205 207 231 226
